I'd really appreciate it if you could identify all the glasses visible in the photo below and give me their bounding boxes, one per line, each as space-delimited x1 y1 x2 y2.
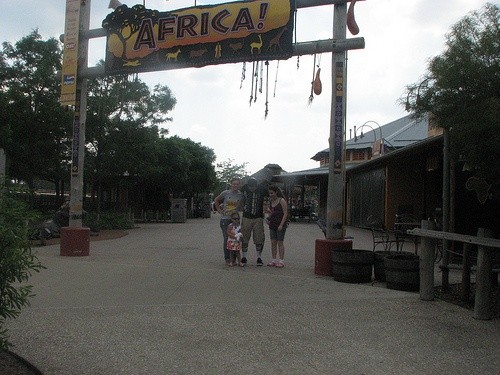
232 218 240 220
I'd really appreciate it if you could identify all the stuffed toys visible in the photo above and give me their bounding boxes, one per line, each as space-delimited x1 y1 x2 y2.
235 232 243 241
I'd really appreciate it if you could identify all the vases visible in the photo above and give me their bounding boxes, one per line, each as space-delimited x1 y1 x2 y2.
383 253 421 292
374 250 414 283
331 248 376 284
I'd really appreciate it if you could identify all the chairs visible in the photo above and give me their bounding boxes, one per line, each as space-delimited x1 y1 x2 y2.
309 213 354 240
392 214 443 264
367 216 405 251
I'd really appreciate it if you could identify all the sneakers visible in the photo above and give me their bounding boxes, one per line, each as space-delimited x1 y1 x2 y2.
240 257 247 267
256 257 263 266
268 261 285 267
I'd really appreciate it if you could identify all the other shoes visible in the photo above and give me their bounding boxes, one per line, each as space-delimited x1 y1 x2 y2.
225 259 236 267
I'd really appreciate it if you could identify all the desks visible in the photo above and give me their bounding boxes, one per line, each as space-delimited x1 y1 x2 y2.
394 222 442 256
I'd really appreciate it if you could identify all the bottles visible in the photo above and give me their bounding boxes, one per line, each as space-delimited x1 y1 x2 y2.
265 212 272 222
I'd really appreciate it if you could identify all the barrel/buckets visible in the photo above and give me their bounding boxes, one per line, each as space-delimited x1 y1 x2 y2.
330 249 375 283
376 251 414 282
383 255 420 293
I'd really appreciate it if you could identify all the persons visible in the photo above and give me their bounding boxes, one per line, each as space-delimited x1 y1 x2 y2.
265 185 289 267
226 211 244 267
240 177 272 266
214 177 244 266
